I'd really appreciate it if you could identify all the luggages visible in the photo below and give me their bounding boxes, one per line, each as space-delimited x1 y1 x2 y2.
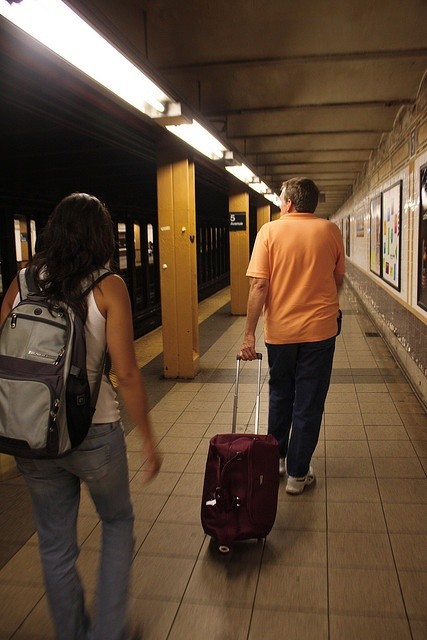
201 351 280 554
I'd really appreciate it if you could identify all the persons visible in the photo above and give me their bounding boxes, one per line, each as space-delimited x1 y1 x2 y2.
0 193 164 640
242 178 345 495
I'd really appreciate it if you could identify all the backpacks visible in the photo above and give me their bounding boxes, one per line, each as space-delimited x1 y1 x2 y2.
2 268 114 461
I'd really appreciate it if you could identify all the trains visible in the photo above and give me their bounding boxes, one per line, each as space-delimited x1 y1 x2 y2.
0 197 232 322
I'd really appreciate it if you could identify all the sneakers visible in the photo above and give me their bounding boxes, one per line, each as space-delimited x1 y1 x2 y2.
286 466 314 495
279 458 286 474
